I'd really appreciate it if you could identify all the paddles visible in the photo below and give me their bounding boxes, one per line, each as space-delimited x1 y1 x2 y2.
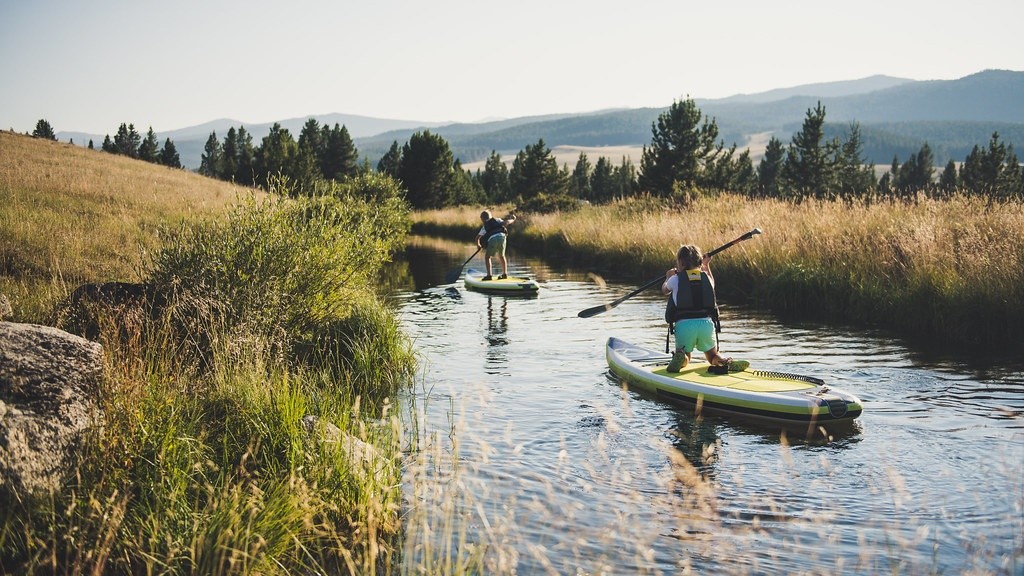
576 227 762 319
443 209 517 284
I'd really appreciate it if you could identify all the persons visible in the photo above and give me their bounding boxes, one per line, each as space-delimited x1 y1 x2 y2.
661 246 750 373
474 210 516 281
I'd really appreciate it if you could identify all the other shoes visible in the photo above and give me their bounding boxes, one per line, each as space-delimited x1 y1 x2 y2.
666 346 685 372
718 356 750 371
498 274 507 279
482 275 492 281
